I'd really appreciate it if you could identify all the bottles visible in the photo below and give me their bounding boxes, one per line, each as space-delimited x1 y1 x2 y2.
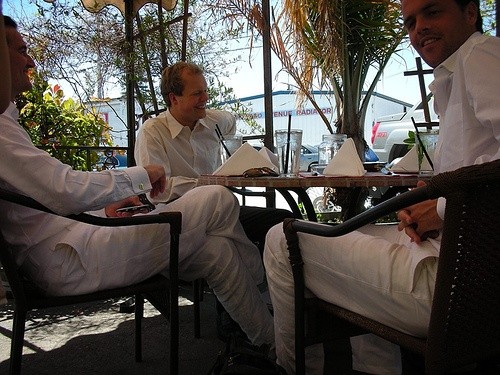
318 134 346 165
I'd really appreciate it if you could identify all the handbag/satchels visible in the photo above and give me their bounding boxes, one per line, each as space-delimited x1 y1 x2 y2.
208 341 287 375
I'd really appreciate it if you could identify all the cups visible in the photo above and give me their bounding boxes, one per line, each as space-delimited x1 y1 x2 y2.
275 129 303 177
414 129 440 175
220 135 242 166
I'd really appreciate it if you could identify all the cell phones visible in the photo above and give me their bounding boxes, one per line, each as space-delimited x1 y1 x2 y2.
116 204 149 212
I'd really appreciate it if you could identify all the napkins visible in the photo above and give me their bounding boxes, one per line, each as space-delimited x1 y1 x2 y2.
259 147 278 168
323 137 367 176
211 142 275 176
391 143 433 171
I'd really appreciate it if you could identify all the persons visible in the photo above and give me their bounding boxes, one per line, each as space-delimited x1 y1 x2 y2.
0 13 275 347
262 0 500 375
134 61 236 204
0 13 11 115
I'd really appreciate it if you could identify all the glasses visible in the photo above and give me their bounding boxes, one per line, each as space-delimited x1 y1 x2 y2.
242 167 280 179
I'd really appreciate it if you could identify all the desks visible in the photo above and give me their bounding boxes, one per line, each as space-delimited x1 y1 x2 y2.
198 172 434 220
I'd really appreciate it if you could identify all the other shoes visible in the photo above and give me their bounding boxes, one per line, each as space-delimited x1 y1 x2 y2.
216 304 274 339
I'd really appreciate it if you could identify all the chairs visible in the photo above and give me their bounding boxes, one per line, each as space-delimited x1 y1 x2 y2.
0 186 182 375
126 153 276 212
282 157 500 375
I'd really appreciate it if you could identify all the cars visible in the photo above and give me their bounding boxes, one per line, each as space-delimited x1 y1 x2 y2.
299 143 319 175
370 89 439 163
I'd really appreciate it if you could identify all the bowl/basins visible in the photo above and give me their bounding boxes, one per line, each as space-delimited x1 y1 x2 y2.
310 164 327 175
362 161 387 172
